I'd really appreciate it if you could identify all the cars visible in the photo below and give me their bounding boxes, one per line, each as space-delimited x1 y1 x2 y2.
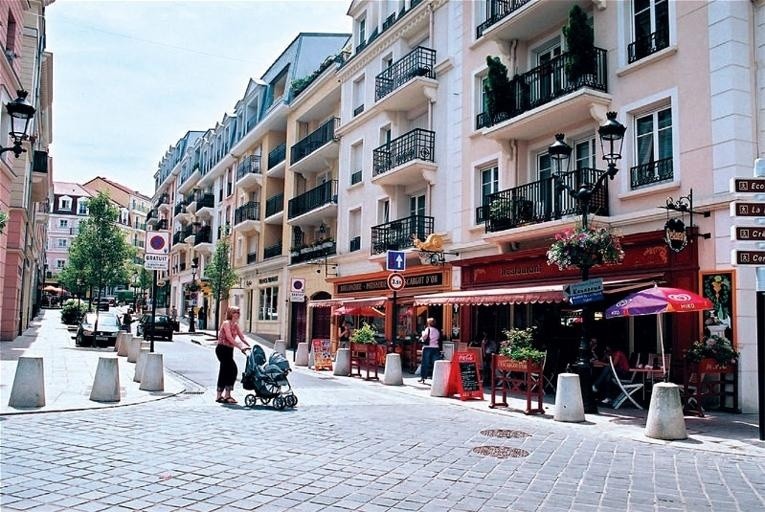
77 310 121 349
136 311 175 341
92 297 110 311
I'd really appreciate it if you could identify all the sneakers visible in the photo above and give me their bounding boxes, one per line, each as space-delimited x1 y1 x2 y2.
602 397 613 403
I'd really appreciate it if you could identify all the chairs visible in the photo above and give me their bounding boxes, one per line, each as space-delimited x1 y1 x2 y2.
608 352 707 412
530 349 559 396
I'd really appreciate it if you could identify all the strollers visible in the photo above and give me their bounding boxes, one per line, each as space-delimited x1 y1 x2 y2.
242 344 298 410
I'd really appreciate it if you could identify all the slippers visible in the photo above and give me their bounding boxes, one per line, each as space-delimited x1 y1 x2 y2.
215 396 237 404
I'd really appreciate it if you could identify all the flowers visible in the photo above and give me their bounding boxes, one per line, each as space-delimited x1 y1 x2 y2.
682 334 739 367
544 206 626 271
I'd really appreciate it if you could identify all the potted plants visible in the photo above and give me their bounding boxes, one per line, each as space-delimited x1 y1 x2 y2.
561 4 597 91
489 199 511 229
489 326 547 416
348 321 379 380
289 236 335 257
482 55 512 125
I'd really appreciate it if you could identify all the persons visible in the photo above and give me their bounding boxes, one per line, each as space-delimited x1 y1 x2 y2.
214 304 252 404
418 317 441 384
337 320 351 348
583 343 630 408
198 306 206 330
170 305 177 322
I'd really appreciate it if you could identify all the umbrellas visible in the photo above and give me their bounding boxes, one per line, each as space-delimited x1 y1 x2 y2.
604 282 713 374
42 285 55 291
53 288 68 292
334 305 385 330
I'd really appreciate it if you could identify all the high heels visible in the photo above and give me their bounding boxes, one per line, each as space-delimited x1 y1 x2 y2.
418 378 425 383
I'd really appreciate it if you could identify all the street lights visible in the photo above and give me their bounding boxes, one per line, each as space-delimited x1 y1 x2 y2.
548 111 627 416
189 264 198 333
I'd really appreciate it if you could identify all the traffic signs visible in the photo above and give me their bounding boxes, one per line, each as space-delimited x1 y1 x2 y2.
563 278 603 297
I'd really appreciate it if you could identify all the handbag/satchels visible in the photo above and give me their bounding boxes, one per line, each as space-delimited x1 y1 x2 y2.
417 336 431 345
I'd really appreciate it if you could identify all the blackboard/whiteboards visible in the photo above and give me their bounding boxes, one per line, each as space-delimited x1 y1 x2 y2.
309 338 333 371
454 351 483 396
